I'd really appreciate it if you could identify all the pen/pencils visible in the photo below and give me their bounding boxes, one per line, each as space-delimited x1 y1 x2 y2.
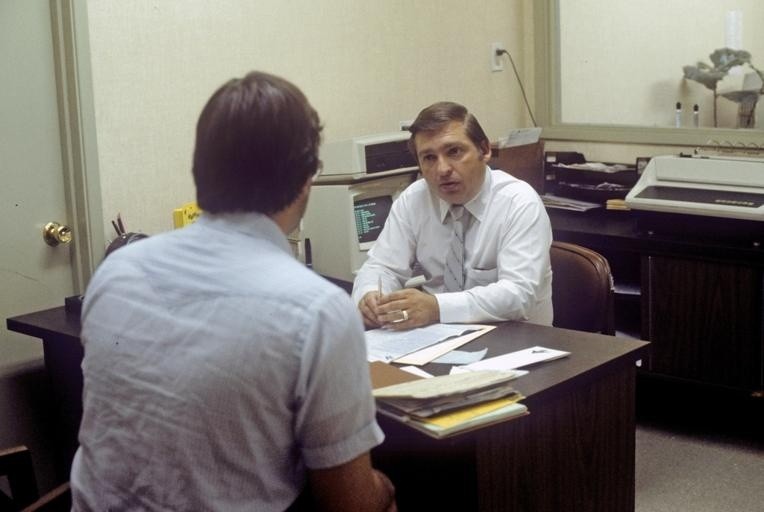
306 238 312 269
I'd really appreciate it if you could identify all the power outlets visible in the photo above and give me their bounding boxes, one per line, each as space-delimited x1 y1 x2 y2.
491 43 503 72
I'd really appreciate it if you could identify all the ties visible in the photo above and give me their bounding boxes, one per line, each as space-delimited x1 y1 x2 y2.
442 205 466 294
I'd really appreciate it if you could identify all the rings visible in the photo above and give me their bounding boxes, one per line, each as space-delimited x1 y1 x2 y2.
403 310 409 320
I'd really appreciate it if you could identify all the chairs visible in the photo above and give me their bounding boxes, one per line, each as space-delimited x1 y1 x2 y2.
548 243 616 337
0 446 70 512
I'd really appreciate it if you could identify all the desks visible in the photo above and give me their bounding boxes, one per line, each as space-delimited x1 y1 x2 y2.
543 205 764 424
5 273 651 511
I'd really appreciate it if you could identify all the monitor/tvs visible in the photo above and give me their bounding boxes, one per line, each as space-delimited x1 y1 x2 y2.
351 189 393 252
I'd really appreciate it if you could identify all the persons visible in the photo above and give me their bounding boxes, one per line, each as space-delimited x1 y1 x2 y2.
345 98 557 329
67 70 404 512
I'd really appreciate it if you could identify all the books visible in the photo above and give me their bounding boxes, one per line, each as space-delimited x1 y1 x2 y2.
355 321 571 441
488 125 544 150
538 190 629 213
